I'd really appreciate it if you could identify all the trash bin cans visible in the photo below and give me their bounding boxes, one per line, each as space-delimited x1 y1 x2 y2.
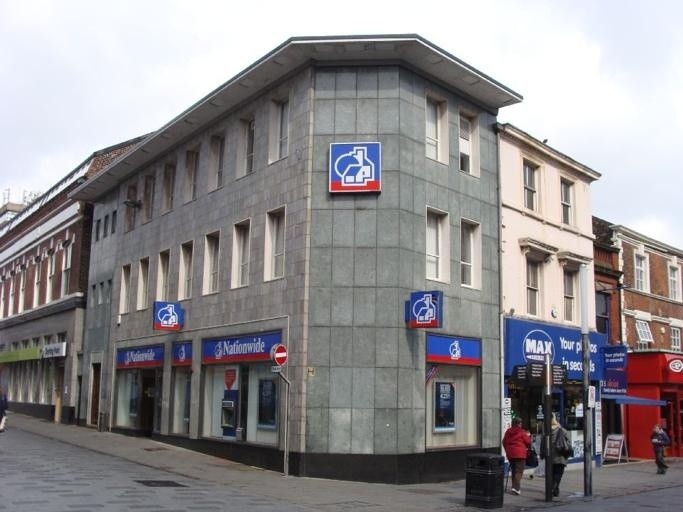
62 406 74 425
464 454 504 509
99 413 109 432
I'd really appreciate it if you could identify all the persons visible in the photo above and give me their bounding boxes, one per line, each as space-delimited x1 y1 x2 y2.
540 411 574 496
500 416 539 495
649 423 670 474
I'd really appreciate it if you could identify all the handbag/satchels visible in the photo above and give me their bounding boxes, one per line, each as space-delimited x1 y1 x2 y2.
556 428 575 460
526 445 539 467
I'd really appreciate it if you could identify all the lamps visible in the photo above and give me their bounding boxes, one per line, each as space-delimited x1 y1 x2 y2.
123 199 143 211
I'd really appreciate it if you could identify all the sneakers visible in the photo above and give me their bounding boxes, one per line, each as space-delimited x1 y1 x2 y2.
511 488 521 495
